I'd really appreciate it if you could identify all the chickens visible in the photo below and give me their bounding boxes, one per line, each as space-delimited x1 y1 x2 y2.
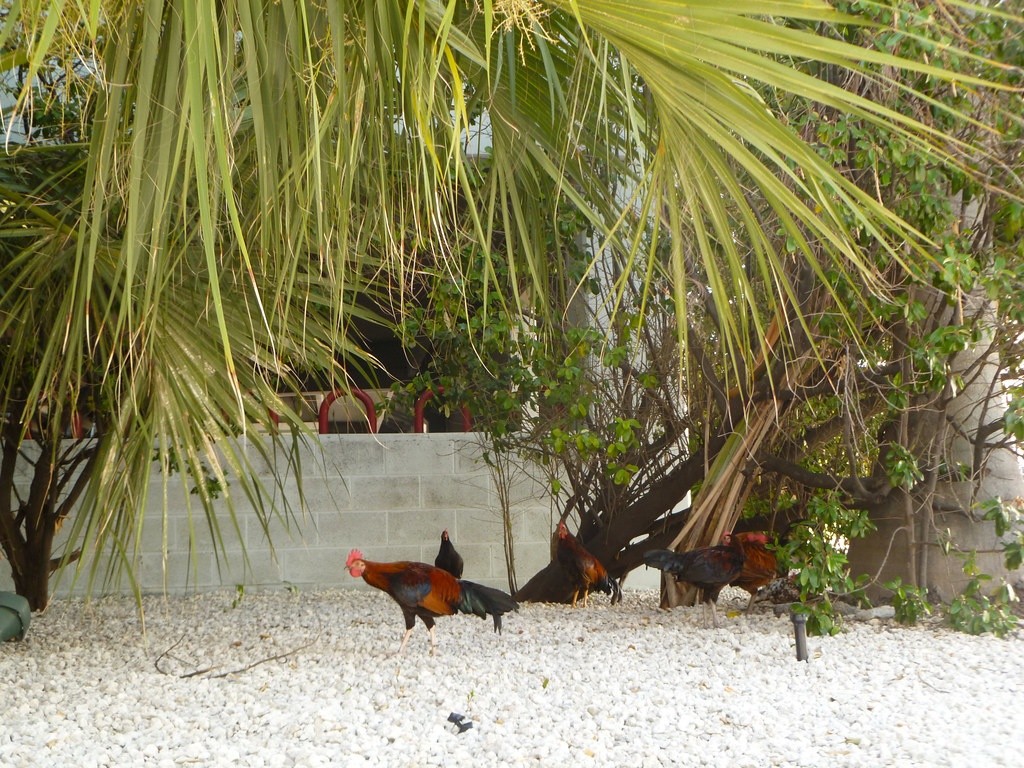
343 529 520 659
645 530 778 629
557 521 622 609
